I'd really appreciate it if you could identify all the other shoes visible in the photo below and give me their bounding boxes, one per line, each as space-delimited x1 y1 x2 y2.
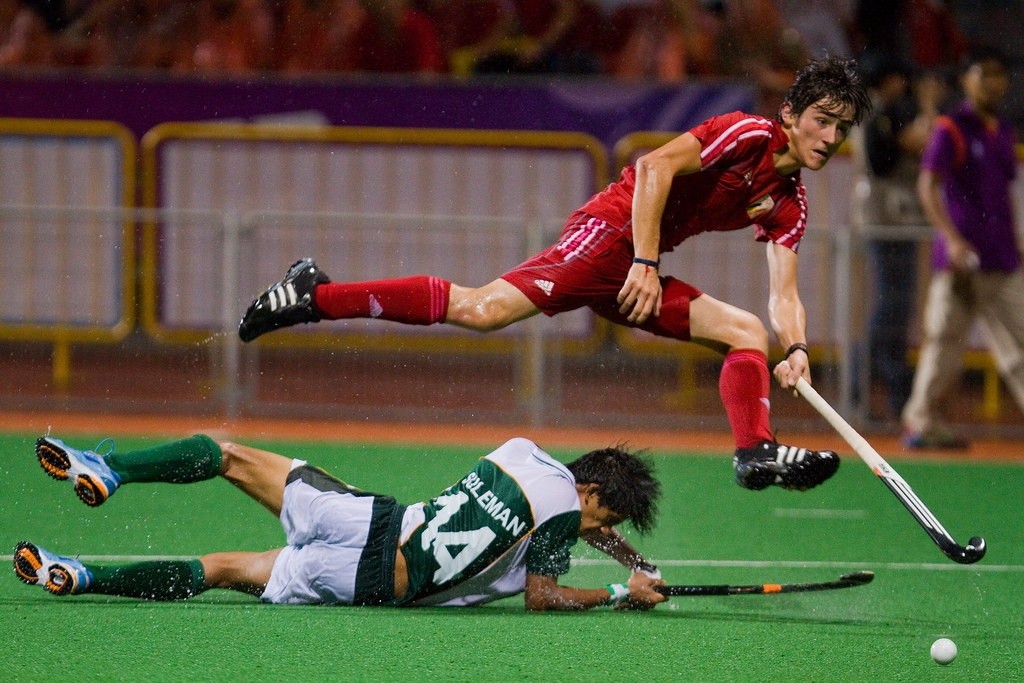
906 420 968 448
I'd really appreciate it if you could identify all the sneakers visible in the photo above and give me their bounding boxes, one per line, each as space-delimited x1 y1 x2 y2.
36 434 122 507
12 540 94 596
731 440 841 492
238 257 332 343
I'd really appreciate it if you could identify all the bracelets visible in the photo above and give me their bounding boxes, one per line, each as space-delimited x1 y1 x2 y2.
633 257 658 269
604 583 630 607
783 342 809 360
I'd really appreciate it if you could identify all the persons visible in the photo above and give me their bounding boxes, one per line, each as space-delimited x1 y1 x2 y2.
238 59 863 491
903 60 1024 451
13 435 670 608
0 0 859 73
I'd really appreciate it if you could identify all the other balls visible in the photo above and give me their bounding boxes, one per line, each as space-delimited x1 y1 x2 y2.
928 636 957 665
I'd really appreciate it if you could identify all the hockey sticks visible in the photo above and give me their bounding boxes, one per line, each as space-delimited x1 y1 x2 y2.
785 362 989 565
649 570 877 597
